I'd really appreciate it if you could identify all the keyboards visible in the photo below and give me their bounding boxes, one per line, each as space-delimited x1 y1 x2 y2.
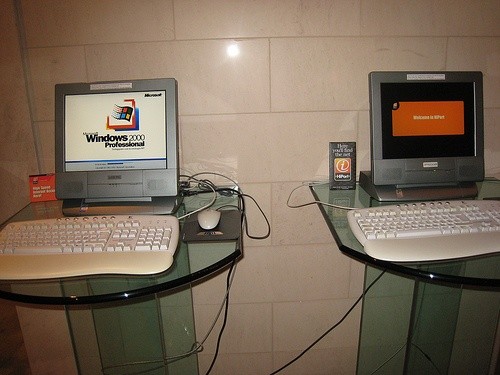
0 215 179 280
347 200 500 262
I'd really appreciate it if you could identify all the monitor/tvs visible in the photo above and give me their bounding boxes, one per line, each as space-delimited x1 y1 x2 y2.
359 71 485 202
55 78 185 215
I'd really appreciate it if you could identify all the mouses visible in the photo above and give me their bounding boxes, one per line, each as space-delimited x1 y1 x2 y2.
197 210 221 229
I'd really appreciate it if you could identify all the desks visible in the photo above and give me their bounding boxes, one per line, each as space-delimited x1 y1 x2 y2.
309 179 500 375
0 185 243 375
